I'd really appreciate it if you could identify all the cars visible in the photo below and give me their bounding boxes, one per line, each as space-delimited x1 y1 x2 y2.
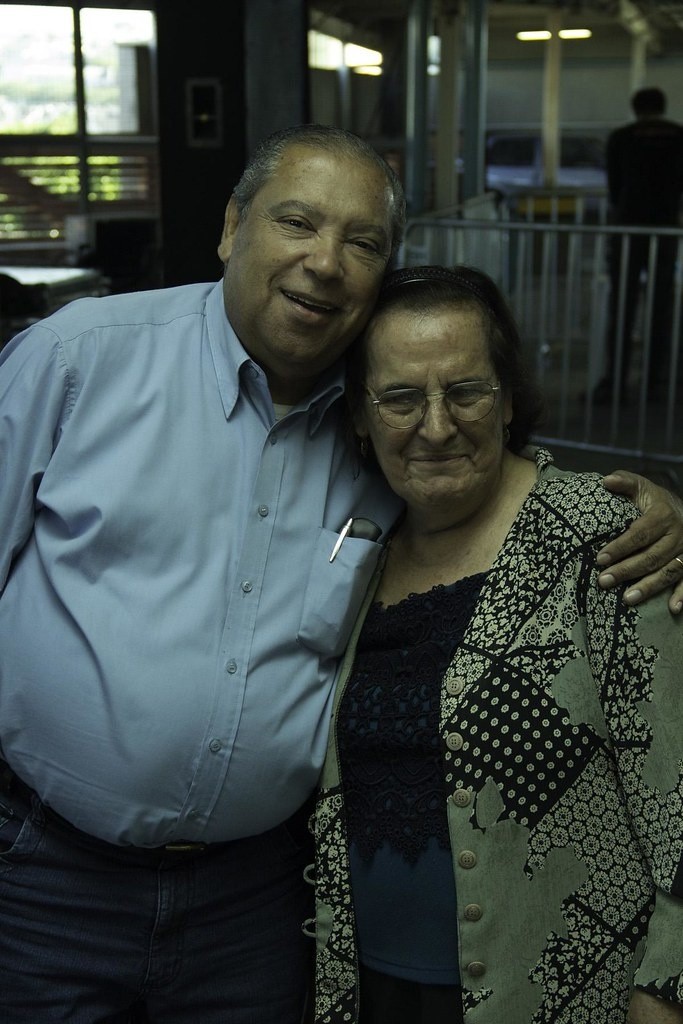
479 129 611 202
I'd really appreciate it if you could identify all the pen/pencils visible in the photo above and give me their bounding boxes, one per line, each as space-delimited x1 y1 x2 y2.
329 518 353 563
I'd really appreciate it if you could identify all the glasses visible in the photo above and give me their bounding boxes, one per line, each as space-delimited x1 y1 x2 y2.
360 380 502 429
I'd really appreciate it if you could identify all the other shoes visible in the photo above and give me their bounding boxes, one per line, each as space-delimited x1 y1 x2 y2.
647 382 670 401
580 381 624 403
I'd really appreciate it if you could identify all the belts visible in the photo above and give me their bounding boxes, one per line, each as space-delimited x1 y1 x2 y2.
0 758 315 870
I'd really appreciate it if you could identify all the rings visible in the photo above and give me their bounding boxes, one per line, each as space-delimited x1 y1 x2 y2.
675 558 683 564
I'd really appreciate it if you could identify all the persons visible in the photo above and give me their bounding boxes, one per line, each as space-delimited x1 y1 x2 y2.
0 124 682 1024
298 266 683 1024
573 85 683 411
